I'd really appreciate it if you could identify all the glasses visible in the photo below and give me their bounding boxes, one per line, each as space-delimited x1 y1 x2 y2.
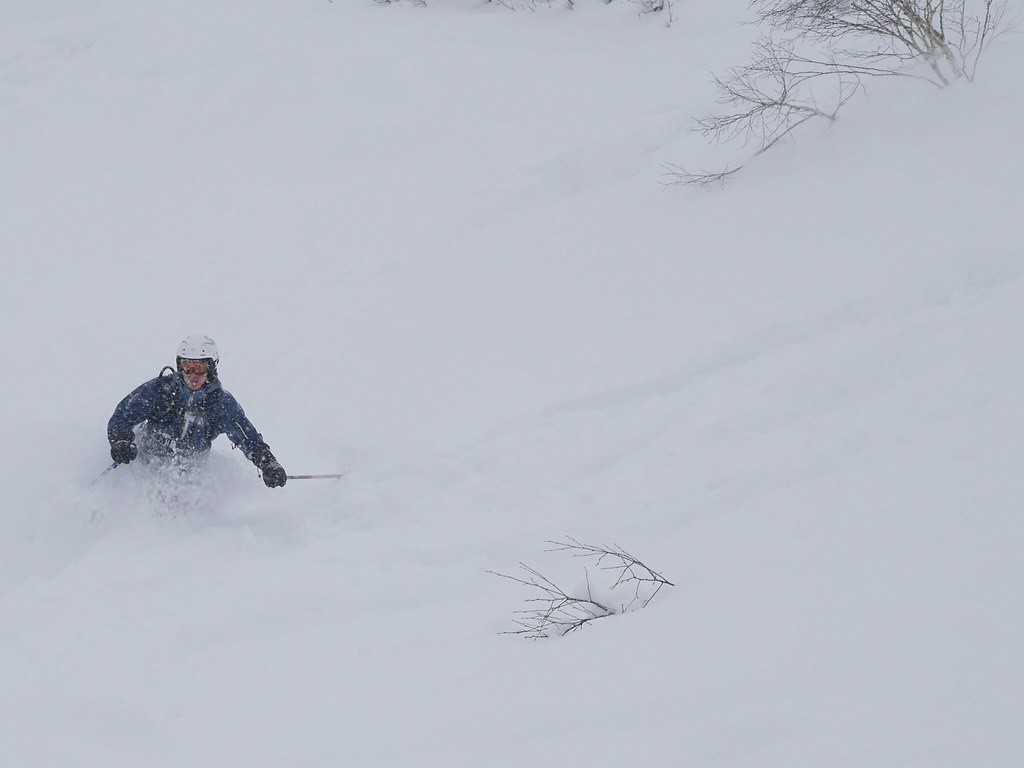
177 359 209 375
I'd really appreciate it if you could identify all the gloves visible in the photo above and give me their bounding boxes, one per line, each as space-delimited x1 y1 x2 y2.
263 462 287 488
111 440 138 466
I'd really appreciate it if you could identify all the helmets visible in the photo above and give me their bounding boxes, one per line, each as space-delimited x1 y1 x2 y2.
176 335 221 363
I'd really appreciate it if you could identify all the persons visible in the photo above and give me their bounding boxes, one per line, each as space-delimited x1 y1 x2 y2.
107 334 287 489
